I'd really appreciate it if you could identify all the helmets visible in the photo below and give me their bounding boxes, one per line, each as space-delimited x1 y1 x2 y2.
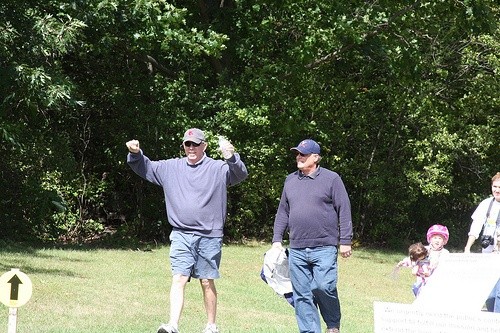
426 225 450 245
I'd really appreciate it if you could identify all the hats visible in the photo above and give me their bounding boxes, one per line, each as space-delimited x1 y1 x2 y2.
290 139 320 155
184 128 206 144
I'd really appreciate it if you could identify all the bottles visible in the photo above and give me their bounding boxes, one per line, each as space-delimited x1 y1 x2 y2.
219 135 233 160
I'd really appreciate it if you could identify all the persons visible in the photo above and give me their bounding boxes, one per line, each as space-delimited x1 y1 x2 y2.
397 224 449 295
465 172 500 253
272 139 353 333
126 128 248 333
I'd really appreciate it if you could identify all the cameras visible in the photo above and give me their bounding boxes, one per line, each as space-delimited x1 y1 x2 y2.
481 236 494 248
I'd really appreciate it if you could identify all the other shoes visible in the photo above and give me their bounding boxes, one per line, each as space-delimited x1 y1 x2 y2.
157 320 180 333
202 322 220 333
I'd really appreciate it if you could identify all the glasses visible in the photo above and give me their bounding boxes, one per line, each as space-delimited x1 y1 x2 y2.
295 151 305 156
184 141 201 147
